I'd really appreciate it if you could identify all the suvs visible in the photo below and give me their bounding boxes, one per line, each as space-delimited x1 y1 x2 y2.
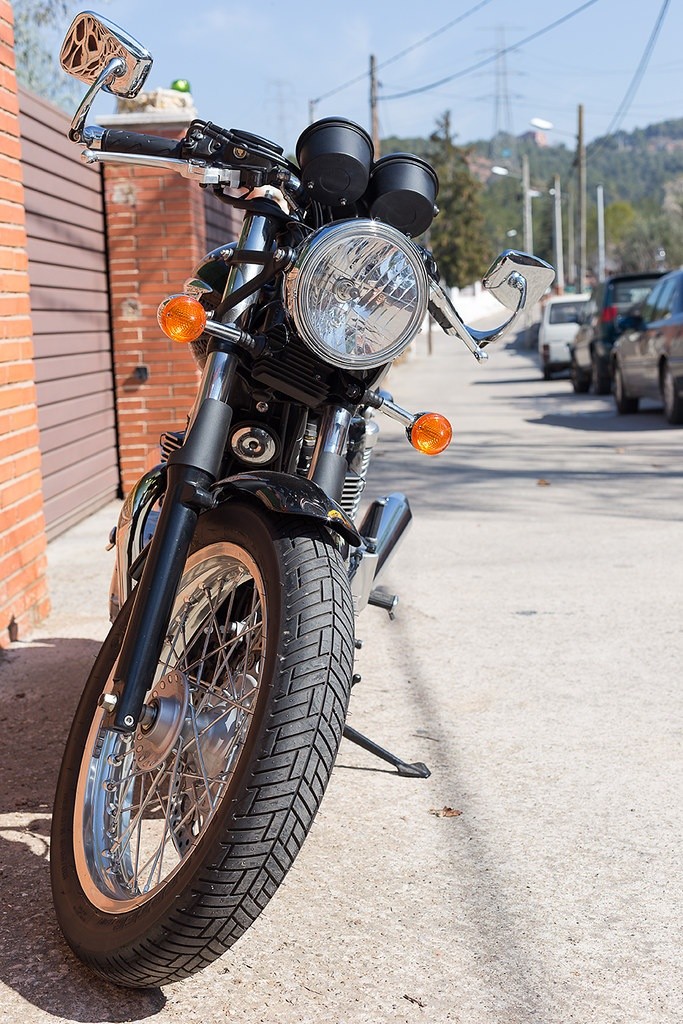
569 271 671 396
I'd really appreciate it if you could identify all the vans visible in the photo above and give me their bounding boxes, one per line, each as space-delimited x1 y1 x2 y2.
538 293 591 380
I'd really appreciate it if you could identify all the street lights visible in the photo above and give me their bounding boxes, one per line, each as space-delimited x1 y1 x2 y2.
528 117 586 291
491 165 577 287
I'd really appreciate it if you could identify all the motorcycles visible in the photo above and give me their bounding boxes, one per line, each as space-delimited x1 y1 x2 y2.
48 9 554 992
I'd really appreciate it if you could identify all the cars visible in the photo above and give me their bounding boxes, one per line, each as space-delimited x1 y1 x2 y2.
608 266 683 425
